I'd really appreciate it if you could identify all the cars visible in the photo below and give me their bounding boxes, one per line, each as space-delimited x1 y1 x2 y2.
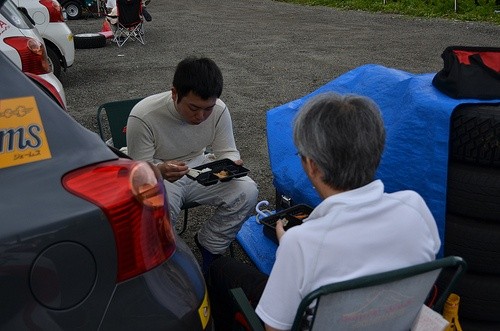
58 0 97 22
0 49 217 331
10 0 76 79
0 0 68 112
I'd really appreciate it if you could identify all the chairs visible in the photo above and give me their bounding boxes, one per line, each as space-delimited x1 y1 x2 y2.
104 0 152 48
97 96 234 255
228 256 476 331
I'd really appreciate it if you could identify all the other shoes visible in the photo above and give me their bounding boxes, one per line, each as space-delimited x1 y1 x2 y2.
194 233 223 273
110 34 124 42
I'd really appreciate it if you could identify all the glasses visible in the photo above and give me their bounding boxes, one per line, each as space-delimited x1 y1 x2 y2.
296 152 305 160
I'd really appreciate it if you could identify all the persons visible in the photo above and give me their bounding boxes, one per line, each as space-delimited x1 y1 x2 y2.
254 93 441 331
127 57 259 272
105 0 145 43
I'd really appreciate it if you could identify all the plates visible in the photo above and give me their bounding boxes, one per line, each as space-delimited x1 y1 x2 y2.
259 203 314 236
185 159 250 186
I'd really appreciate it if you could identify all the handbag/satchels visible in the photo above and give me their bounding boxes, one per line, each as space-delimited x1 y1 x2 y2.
431 45 500 101
102 21 113 38
